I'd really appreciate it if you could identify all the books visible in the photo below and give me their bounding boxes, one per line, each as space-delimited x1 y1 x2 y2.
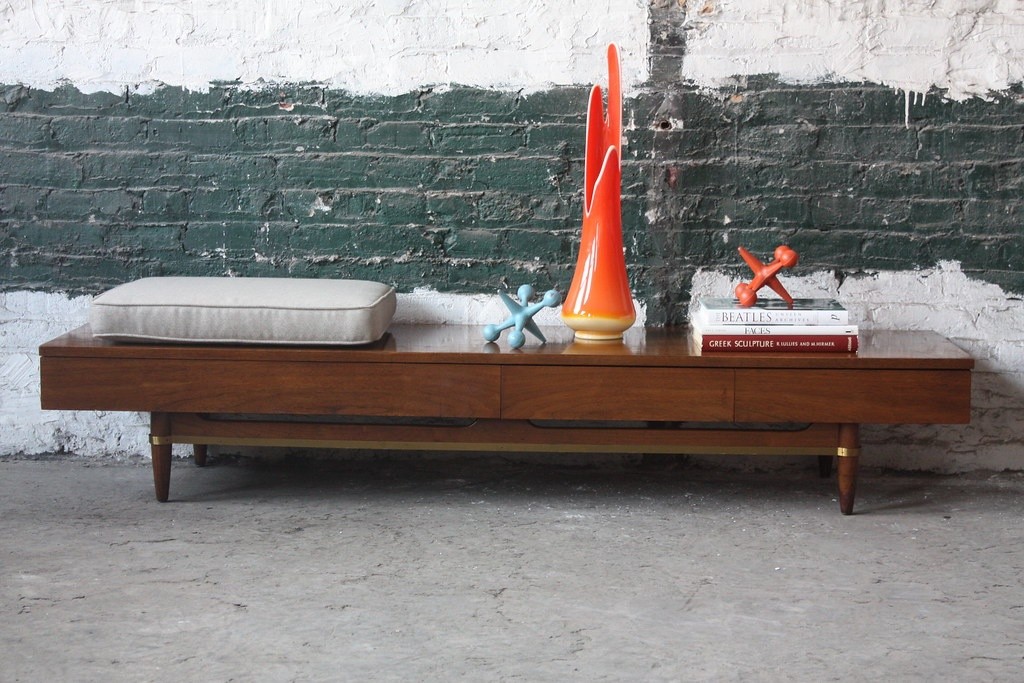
688 297 860 354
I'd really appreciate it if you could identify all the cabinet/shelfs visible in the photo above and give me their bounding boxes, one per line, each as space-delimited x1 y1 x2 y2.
39 322 976 516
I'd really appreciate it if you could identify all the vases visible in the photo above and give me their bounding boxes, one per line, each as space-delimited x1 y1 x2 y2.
562 43 635 342
